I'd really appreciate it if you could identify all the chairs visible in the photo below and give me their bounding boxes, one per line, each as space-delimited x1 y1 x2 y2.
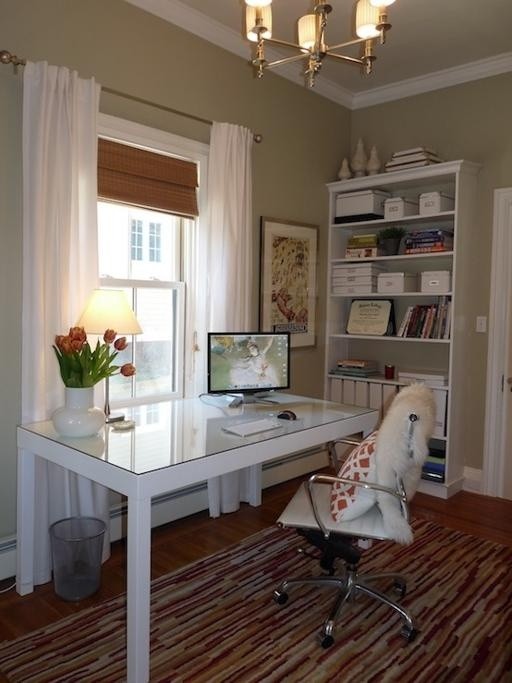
273 384 436 651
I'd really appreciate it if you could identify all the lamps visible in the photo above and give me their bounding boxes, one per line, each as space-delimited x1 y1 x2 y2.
72 289 142 423
243 0 396 87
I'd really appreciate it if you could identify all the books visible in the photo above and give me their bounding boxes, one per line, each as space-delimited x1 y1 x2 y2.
397 296 451 339
397 370 448 386
328 358 381 379
401 227 453 254
384 145 445 173
421 450 445 483
344 232 385 258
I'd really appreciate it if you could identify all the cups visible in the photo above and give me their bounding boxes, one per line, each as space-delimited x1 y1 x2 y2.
385 364 395 380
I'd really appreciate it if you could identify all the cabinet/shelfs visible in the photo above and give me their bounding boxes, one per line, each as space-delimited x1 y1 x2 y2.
324 160 482 500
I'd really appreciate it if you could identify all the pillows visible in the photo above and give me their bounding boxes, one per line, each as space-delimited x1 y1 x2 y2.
330 427 383 523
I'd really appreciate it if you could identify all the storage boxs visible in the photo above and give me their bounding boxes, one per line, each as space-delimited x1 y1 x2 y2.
383 198 417 218
421 271 452 293
335 190 391 214
378 271 418 293
419 191 454 215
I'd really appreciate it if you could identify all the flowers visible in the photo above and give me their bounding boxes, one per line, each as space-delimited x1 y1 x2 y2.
51 326 137 387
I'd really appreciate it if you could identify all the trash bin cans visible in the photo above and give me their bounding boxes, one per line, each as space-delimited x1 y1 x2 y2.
48 516 107 602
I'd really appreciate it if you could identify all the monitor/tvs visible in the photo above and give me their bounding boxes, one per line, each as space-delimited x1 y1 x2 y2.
205 331 291 410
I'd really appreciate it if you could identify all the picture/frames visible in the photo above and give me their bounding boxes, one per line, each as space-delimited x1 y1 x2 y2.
259 217 320 349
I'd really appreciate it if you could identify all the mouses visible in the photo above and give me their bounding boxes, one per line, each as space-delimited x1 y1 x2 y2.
277 410 297 420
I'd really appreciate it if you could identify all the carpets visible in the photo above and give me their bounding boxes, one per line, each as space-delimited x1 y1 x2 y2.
0 519 512 683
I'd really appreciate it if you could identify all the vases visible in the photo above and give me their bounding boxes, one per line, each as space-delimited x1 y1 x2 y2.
52 387 106 438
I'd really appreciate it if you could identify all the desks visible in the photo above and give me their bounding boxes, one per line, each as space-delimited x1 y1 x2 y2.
15 388 379 683
